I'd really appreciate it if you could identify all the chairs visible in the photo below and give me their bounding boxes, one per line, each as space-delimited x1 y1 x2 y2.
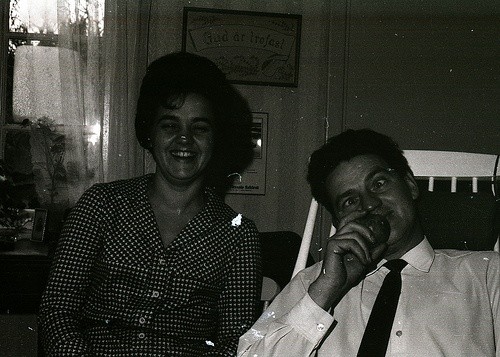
290 149 500 279
38 275 282 357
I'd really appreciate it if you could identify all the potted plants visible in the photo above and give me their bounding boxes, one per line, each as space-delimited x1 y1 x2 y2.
21 117 67 236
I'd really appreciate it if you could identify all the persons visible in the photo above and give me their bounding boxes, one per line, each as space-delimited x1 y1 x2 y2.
33 50 262 357
235 129 500 356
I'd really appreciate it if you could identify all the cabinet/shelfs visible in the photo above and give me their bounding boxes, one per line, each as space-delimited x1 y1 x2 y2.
0 239 52 316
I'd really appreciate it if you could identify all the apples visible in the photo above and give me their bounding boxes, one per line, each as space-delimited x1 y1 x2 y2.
355 214 391 249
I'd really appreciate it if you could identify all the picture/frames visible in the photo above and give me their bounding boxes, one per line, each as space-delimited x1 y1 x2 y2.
215 112 269 196
30 208 48 243
181 6 302 88
